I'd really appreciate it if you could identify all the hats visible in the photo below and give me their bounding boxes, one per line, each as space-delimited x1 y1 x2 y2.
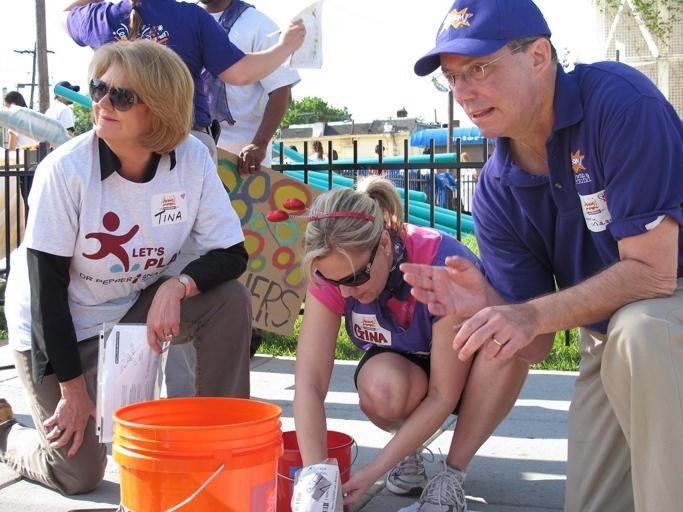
54 81 81 96
414 0 551 77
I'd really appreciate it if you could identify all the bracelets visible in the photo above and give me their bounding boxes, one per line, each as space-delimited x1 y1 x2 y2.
175 274 191 302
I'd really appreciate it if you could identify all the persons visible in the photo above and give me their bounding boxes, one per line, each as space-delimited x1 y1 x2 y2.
292 174 530 512
45 81 80 151
200 0 301 176
63 0 307 171
398 1 683 512
0 37 252 497
3 91 48 229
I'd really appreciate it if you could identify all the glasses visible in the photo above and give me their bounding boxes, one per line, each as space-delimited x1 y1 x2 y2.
89 78 145 112
314 229 383 287
431 44 524 94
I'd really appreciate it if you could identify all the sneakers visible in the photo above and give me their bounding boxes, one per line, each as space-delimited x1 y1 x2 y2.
386 446 429 498
417 460 468 512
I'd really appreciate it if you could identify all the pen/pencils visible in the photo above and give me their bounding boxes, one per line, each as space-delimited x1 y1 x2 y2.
267 27 287 37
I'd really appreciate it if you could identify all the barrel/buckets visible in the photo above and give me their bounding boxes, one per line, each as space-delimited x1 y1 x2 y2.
278 431 358 512
113 396 282 512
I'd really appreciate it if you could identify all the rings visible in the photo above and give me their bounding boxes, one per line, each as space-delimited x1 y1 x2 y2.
158 337 165 340
166 334 173 339
493 337 504 347
249 165 255 169
54 425 62 434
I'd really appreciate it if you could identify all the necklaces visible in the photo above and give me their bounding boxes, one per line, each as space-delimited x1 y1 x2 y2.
520 140 548 168
